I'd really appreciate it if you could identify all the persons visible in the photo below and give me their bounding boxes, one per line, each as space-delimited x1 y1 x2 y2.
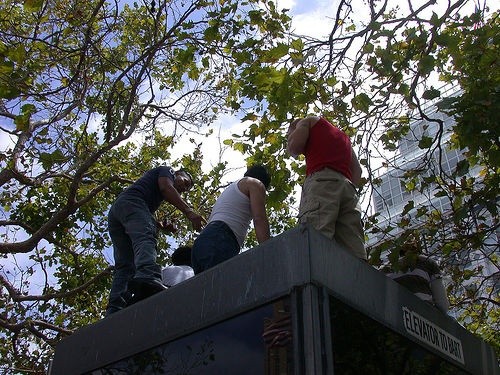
104 166 209 319
161 245 196 288
286 115 370 262
190 164 272 277
